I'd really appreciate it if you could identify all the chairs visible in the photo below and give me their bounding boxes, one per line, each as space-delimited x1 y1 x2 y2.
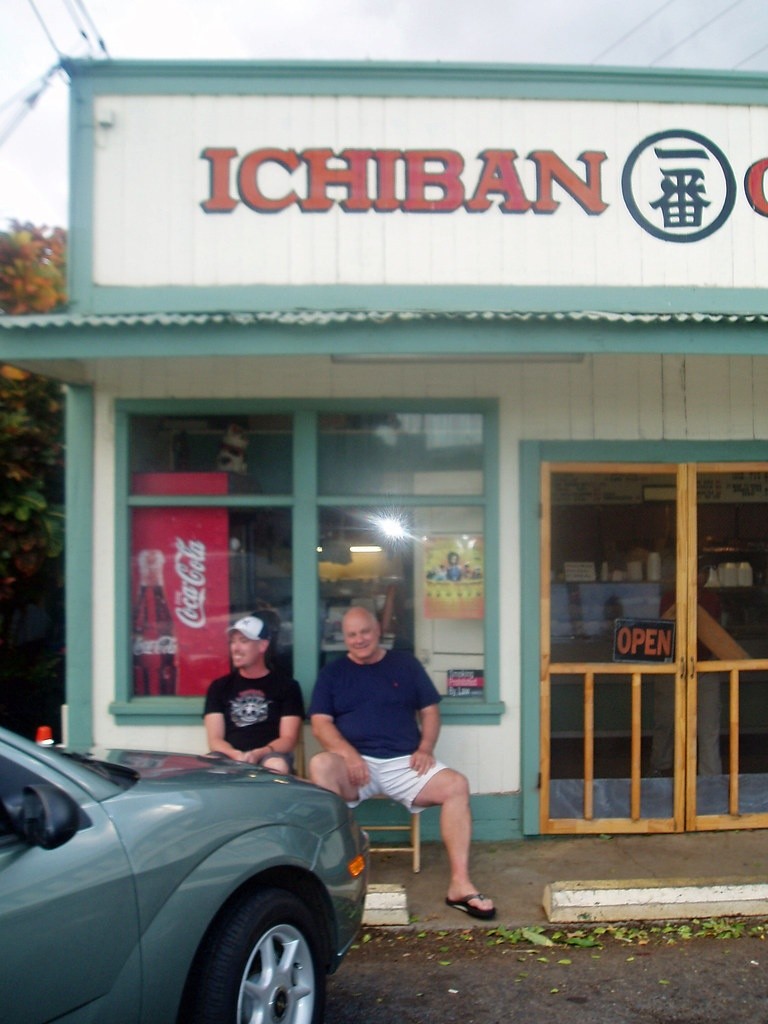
349 795 421 873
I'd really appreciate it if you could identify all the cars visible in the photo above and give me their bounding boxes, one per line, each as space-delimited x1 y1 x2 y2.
1 729 369 1024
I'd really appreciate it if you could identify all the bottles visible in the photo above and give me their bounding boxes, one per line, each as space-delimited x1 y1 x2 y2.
646 548 661 580
134 549 176 696
610 566 624 581
600 557 608 582
705 559 753 587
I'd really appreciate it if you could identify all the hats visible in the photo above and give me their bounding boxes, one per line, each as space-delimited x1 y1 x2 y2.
226 614 274 640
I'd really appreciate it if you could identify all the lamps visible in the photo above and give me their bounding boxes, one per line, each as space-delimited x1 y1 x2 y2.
330 353 583 362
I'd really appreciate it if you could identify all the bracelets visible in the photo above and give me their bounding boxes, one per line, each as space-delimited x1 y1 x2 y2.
265 744 275 754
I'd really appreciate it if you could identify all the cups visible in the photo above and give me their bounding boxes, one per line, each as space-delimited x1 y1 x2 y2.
627 560 643 581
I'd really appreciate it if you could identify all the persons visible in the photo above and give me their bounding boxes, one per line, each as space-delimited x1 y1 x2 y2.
204 616 302 774
308 607 496 919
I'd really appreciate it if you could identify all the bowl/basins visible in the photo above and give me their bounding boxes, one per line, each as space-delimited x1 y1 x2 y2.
350 545 382 578
318 562 348 582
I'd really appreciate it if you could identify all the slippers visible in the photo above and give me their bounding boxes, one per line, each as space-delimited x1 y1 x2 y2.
445 893 497 917
